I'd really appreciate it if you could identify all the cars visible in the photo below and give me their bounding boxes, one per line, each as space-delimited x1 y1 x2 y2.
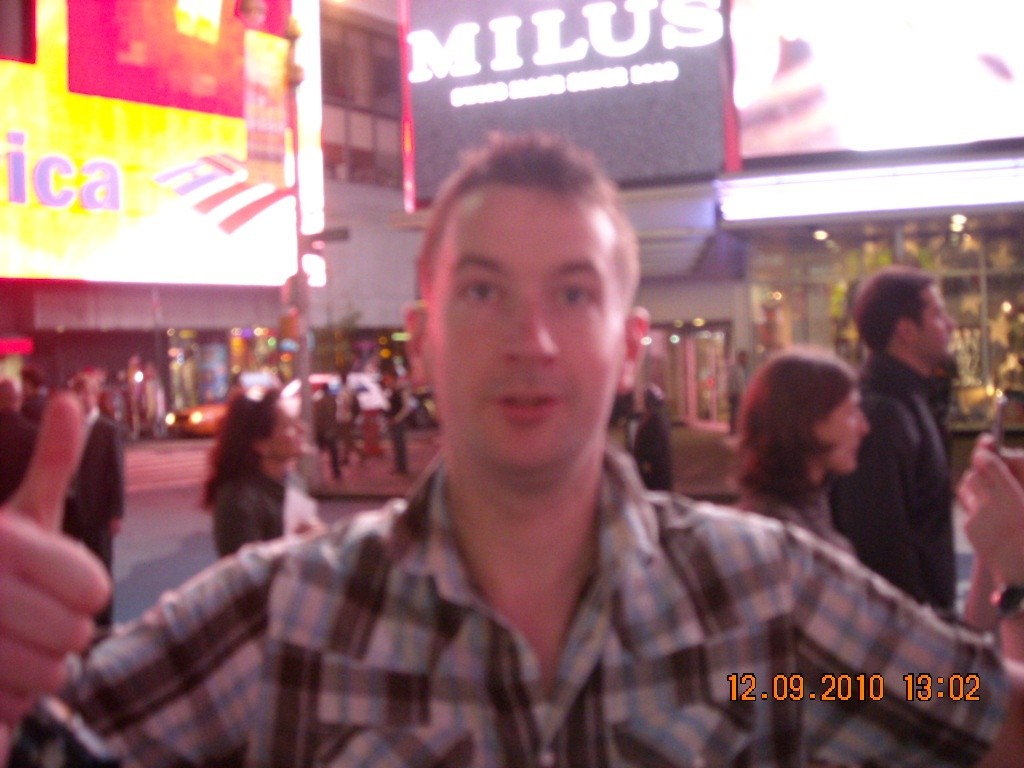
164 373 437 430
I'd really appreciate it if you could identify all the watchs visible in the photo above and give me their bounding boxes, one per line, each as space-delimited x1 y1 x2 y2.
990 584 1024 616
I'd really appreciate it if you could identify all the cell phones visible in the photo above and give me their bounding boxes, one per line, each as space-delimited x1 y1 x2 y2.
992 395 1007 456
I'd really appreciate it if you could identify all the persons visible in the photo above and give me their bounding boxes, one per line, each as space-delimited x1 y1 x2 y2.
0 135 1023 768
